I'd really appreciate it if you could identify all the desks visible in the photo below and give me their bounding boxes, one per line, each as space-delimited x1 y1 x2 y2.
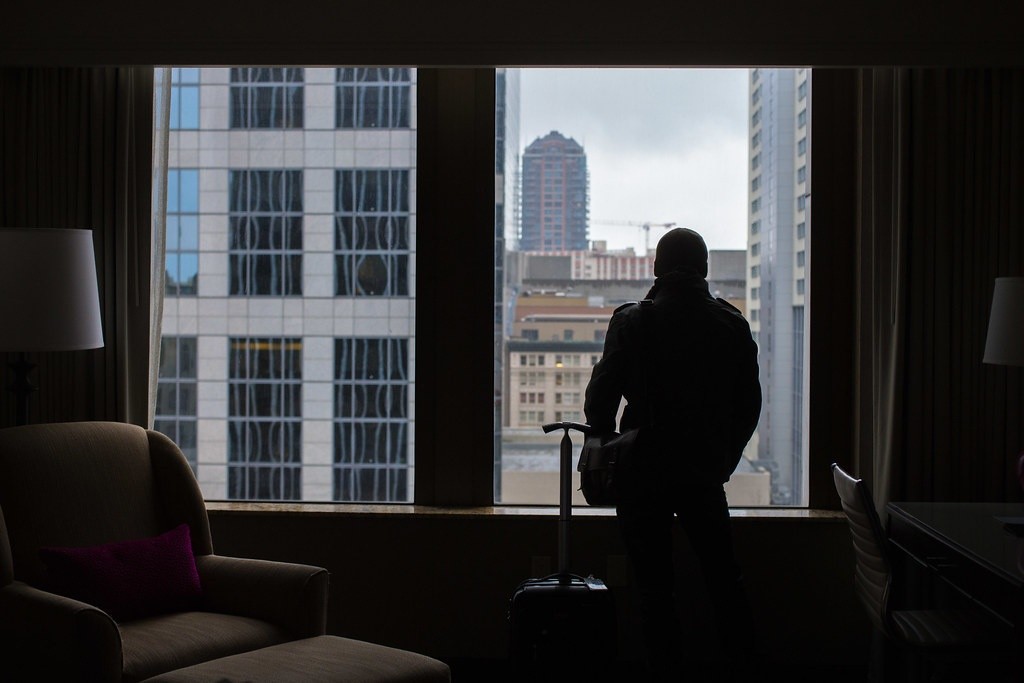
885 501 1024 638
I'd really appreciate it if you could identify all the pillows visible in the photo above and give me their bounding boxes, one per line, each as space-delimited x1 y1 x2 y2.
45 525 204 616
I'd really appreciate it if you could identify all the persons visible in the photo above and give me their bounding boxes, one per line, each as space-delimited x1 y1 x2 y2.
584 227 762 683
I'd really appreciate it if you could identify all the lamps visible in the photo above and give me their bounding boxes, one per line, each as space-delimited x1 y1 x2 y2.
0 228 105 425
982 278 1024 372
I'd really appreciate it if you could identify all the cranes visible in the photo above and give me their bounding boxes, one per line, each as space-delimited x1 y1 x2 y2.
593 221 677 253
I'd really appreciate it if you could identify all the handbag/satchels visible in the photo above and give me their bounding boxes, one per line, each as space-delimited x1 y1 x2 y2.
578 425 649 506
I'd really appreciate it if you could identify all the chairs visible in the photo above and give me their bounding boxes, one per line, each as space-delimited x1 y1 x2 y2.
0 421 329 683
829 462 1013 683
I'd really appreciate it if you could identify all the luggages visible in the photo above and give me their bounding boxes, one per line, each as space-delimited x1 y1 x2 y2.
503 423 615 683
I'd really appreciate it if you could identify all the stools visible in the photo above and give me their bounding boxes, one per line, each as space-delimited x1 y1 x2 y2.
138 632 452 683
